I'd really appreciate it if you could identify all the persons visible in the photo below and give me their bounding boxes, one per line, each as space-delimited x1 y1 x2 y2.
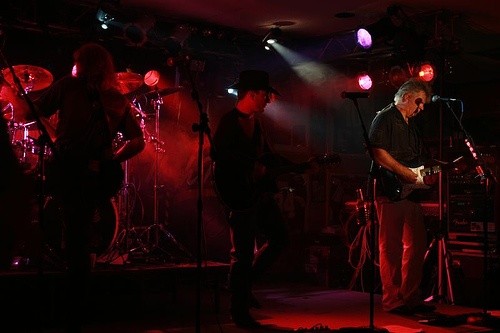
20 42 146 271
365 79 436 316
210 71 320 329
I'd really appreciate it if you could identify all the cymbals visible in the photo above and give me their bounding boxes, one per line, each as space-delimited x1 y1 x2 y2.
111 71 142 94
2 65 53 91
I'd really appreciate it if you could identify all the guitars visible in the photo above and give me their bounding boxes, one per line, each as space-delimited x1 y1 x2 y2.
212 152 341 212
379 152 468 201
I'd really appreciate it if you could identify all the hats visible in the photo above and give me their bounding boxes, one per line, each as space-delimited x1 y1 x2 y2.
227 69 282 96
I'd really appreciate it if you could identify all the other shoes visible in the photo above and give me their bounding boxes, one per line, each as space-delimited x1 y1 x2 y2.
387 300 435 316
237 294 272 332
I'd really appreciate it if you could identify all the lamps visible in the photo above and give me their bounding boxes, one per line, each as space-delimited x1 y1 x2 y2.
93 4 452 100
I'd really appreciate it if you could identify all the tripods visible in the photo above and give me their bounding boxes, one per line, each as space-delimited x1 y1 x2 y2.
137 100 192 261
423 107 458 303
108 157 149 262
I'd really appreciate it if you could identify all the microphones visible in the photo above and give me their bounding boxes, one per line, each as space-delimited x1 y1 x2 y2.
342 91 369 100
431 94 460 103
